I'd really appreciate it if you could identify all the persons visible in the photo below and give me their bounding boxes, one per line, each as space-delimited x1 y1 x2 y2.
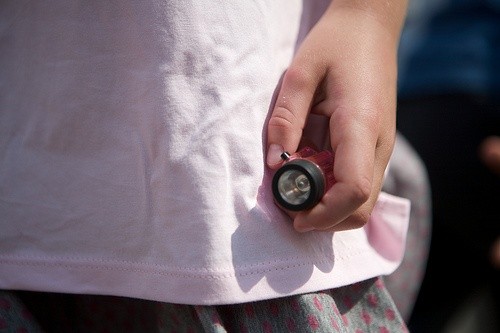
396 0 500 333
0 0 431 333
